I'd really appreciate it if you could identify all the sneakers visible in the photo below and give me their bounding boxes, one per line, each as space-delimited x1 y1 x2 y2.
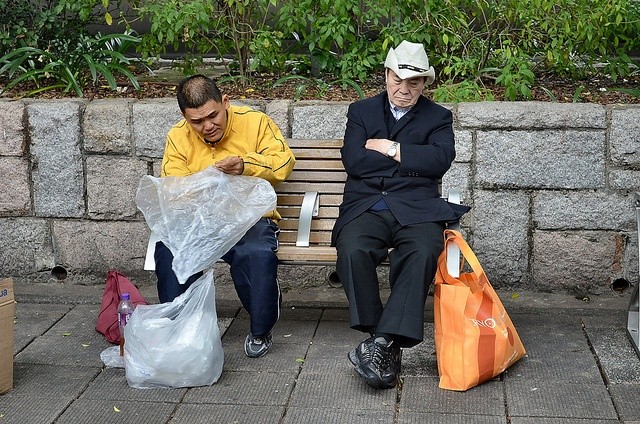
348 336 402 389
245 332 271 358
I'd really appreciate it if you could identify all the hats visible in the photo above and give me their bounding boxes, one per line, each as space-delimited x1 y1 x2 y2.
384 40 435 86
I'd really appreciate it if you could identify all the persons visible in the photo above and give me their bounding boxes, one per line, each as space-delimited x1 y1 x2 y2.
331 40 470 387
154 75 295 356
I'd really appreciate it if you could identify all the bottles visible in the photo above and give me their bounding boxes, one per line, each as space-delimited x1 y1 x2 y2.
117 292 136 367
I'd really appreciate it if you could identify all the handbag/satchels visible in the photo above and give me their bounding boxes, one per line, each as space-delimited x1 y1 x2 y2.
433 229 526 391
94 270 148 346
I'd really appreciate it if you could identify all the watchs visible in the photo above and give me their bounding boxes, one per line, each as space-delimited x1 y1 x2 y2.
385 142 398 157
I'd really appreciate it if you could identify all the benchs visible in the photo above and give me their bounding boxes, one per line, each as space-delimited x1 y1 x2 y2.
143 138 461 278
